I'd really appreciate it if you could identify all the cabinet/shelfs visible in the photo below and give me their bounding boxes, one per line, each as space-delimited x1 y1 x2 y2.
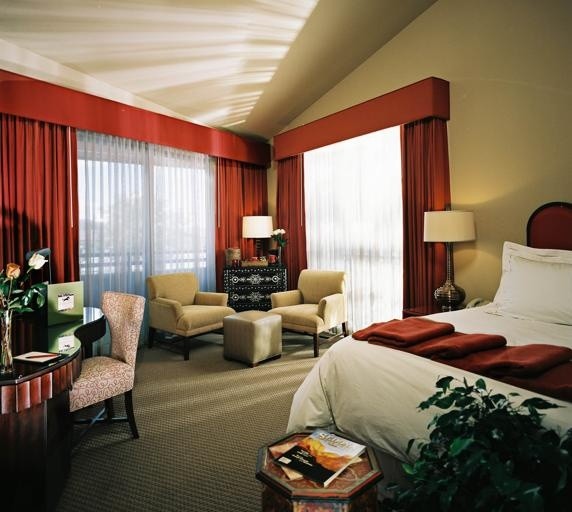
223 266 287 312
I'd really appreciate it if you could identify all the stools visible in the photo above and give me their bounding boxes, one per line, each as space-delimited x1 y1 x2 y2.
255 430 383 512
223 310 282 368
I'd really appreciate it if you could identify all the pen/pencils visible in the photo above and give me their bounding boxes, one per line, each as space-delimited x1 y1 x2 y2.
25 354 57 358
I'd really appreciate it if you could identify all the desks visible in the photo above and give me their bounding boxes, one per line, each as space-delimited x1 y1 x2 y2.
0 306 106 512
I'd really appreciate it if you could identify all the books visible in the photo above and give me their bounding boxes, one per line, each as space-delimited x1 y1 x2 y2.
273 427 367 487
269 441 364 480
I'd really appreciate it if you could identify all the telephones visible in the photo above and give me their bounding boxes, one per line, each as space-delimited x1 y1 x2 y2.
465 298 489 309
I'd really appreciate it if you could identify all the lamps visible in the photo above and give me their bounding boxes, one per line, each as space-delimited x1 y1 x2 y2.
424 211 476 312
243 216 273 261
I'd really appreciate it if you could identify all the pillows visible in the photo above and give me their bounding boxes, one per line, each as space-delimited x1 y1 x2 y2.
484 241 572 326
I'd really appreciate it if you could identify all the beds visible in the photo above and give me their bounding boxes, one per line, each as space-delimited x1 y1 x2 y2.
330 201 572 512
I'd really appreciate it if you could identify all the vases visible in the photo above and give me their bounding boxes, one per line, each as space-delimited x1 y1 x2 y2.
0 309 16 374
277 246 282 268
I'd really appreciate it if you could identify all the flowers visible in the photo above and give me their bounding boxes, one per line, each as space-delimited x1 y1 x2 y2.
271 229 287 247
0 248 51 372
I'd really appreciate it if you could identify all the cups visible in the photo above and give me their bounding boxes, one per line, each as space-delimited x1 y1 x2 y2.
232 254 278 268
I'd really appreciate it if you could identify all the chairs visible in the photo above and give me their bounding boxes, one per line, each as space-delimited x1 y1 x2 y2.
147 272 236 360
268 269 349 358
68 291 146 457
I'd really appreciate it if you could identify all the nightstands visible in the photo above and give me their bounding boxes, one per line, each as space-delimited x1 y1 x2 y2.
403 305 469 321
